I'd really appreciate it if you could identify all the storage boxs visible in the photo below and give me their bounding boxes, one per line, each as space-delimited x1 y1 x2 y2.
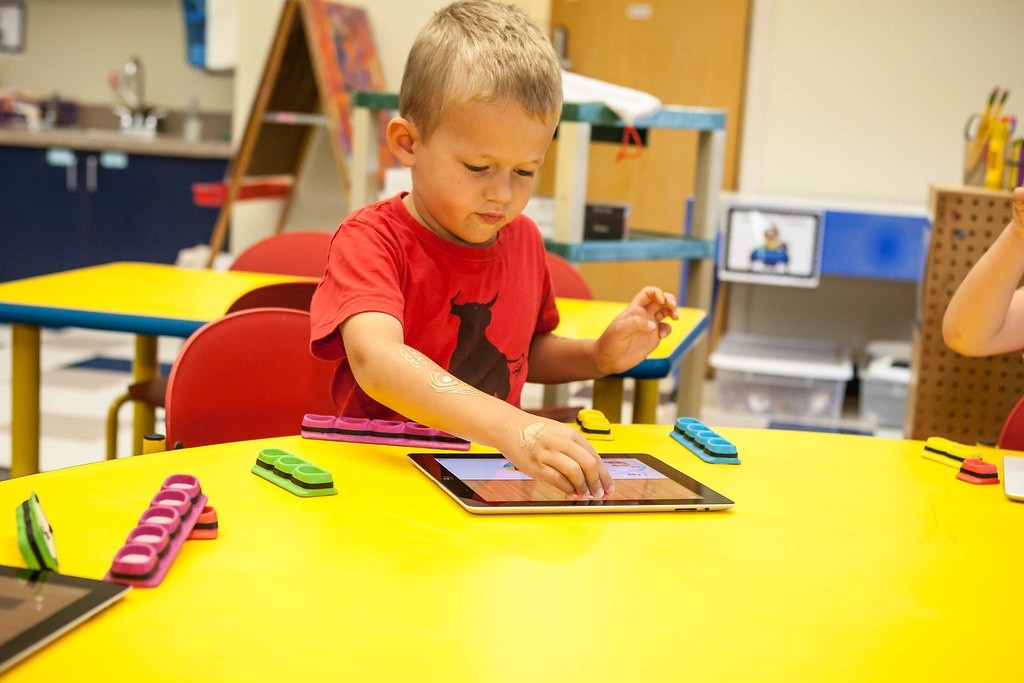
855 338 914 429
708 332 856 424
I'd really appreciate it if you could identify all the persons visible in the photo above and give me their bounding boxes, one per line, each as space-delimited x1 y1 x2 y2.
942 185 1024 357
310 0 678 497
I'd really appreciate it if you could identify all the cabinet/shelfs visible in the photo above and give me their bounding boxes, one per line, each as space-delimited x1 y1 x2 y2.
345 92 724 433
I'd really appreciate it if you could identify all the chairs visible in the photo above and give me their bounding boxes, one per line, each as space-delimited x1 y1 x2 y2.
110 224 339 464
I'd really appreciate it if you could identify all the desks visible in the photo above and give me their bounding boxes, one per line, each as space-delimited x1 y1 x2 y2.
0 125 233 181
0 262 707 482
0 422 1022 683
